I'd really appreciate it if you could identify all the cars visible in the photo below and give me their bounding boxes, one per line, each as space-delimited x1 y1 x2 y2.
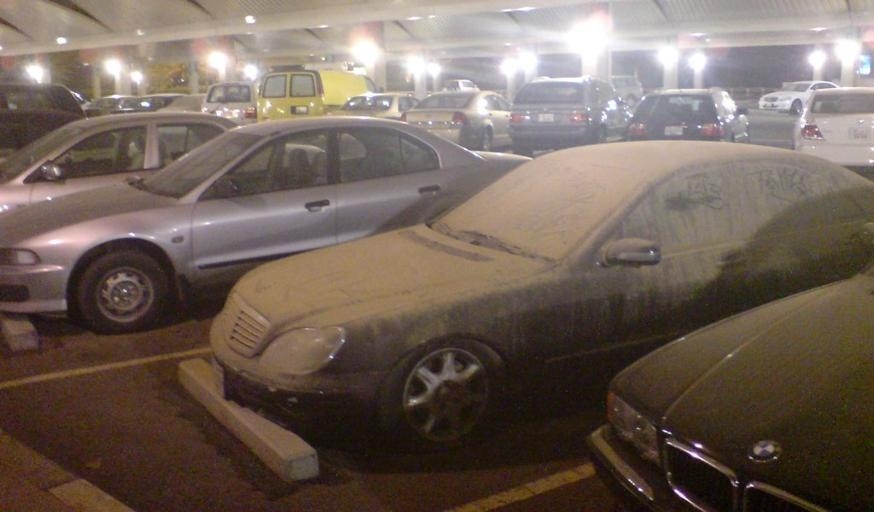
0 71 479 149
0 112 243 214
793 87 874 167
0 114 534 333
510 75 750 156
586 268 874 512
759 80 840 115
402 89 514 153
211 140 874 443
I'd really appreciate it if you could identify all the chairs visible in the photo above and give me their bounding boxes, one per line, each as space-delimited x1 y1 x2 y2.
127 136 145 171
274 148 310 189
309 152 330 189
